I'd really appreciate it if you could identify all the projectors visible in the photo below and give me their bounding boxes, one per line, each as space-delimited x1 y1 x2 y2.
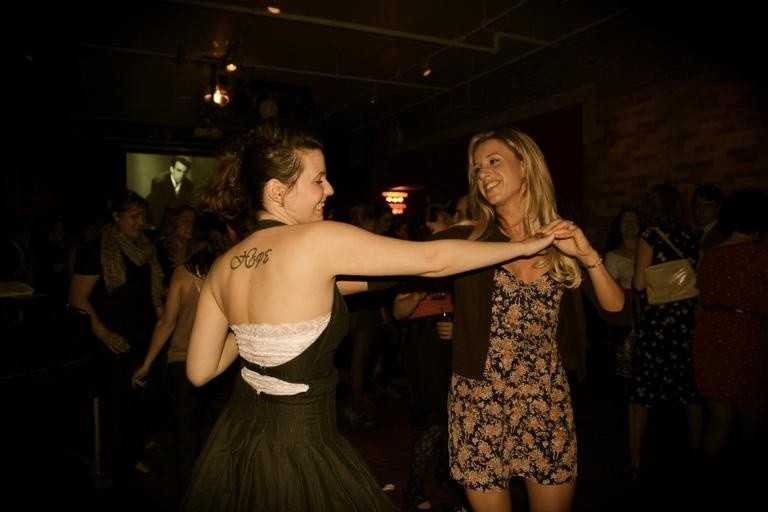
193 127 223 139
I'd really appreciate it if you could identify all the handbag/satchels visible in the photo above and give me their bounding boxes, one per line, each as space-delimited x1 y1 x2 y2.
643 227 701 306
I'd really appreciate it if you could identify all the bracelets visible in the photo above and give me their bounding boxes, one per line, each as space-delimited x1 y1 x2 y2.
583 252 603 269
232 332 239 355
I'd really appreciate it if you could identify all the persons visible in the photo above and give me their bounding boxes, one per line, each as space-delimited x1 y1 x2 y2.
143 154 197 229
336 127 624 512
182 121 575 511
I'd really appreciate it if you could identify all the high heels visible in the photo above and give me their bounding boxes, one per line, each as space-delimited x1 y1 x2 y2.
344 408 375 428
